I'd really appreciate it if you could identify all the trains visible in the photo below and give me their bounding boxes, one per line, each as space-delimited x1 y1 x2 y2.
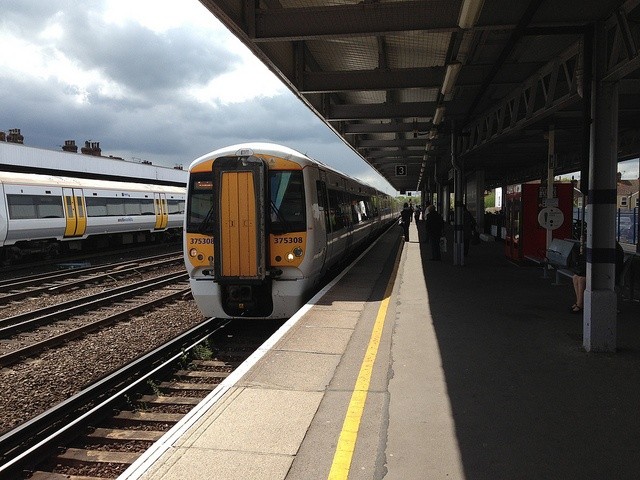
182 139 403 320
0 140 189 267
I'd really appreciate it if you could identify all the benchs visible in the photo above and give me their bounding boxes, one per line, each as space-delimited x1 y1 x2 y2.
551 238 634 287
523 237 579 286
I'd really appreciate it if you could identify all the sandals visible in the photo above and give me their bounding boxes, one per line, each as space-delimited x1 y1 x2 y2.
568 303 583 313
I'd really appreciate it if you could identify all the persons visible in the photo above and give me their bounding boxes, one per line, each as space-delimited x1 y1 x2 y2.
425 205 445 261
567 235 624 314
463 205 477 257
401 202 411 242
409 202 414 222
423 201 431 243
415 204 424 227
